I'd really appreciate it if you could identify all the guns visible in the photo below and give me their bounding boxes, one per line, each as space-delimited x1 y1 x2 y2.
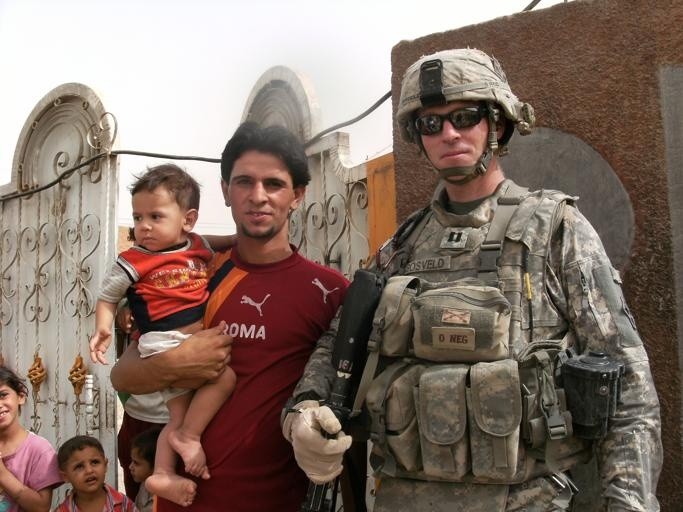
299 266 388 511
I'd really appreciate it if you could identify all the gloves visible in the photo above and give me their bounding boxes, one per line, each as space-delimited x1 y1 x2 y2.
282 399 352 485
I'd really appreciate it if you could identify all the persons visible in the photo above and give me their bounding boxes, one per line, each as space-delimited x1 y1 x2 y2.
52 434 138 512
87 161 239 507
277 45 667 511
109 119 353 511
128 427 161 511
1 363 66 512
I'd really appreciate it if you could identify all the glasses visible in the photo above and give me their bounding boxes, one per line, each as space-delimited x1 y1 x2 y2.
414 105 488 135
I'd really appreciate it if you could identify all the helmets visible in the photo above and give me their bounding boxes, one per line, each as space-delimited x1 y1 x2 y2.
396 48 535 144
